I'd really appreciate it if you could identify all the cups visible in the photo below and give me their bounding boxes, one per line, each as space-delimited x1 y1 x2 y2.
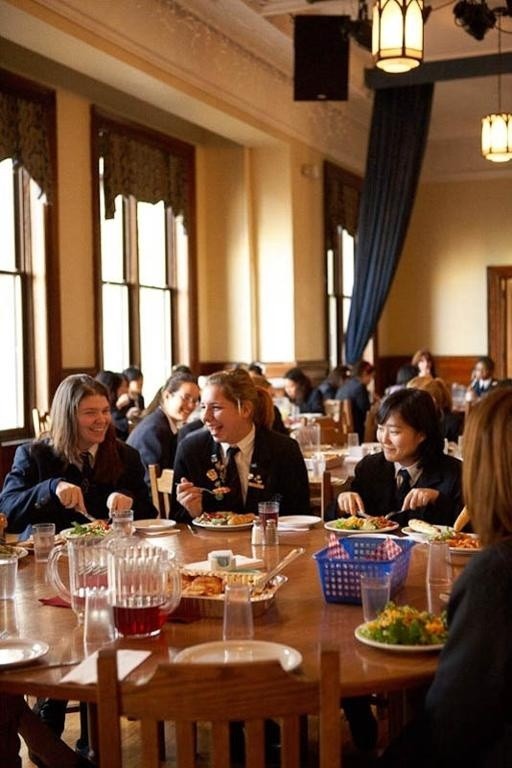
32 522 55 564
84 587 118 643
427 539 453 586
113 509 134 535
294 424 363 483
2 549 17 599
47 536 110 627
224 581 254 639
357 571 393 620
107 545 183 635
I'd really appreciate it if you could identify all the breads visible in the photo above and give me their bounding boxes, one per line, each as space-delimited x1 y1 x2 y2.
164 575 222 598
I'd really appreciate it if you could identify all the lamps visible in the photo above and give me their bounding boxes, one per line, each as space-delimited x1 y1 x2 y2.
371 0 428 76
481 8 512 162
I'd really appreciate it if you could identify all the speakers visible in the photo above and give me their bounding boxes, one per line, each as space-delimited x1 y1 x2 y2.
293 13 351 103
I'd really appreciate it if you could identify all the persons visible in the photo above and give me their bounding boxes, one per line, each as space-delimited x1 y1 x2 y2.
324 388 476 768
168 367 310 768
374 385 512 768
95 350 512 519
1 373 159 768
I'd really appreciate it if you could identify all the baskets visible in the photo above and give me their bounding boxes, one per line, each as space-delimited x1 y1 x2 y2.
312 538 415 604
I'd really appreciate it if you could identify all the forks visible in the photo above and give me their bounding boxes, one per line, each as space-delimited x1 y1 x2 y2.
252 545 308 594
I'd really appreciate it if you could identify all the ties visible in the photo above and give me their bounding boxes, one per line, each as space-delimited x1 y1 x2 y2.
398 470 411 491
224 447 244 507
79 452 92 472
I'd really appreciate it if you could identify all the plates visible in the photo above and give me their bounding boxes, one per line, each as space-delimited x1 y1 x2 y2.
172 639 304 673
134 518 178 532
324 517 400 535
192 516 260 532
402 525 456 537
278 515 323 528
353 619 447 652
0 634 50 667
2 545 28 561
426 532 483 554
174 566 288 617
59 520 138 539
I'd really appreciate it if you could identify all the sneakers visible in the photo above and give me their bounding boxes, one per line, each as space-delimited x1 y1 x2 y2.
344 704 379 750
